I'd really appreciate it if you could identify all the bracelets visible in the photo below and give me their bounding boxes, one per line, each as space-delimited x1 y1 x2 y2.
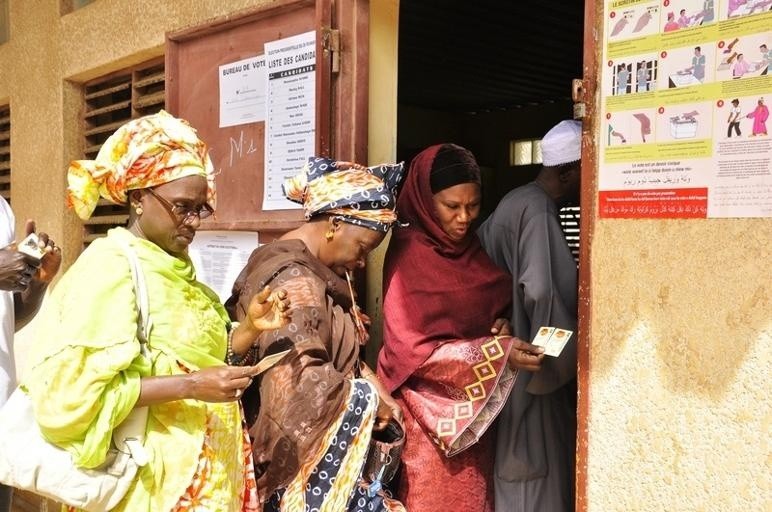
225 327 253 366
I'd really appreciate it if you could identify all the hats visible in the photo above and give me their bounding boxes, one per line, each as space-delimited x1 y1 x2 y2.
430 144 481 194
539 118 582 168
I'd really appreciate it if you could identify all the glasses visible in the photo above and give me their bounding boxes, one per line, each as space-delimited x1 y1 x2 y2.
146 188 214 226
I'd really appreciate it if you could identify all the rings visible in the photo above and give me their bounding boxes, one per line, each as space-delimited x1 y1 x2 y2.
234 389 243 399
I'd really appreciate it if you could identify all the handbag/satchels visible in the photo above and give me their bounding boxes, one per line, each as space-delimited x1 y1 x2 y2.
362 415 405 484
0 241 151 512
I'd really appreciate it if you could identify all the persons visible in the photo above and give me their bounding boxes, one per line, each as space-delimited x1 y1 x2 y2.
30 111 292 512
0 170 63 511
223 158 411 512
480 117 580 512
377 142 544 512
